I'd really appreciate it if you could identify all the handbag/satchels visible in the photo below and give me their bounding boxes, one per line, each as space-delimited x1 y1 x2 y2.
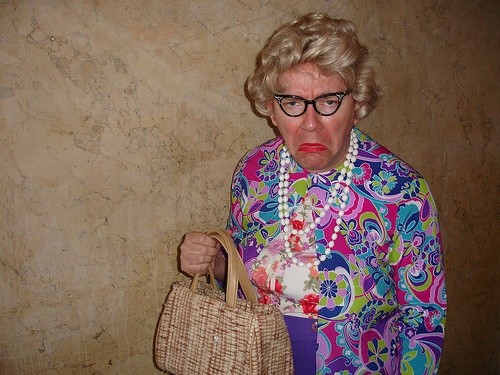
155 229 294 375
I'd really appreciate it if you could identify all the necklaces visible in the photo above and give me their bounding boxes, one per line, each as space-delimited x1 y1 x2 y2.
265 128 358 268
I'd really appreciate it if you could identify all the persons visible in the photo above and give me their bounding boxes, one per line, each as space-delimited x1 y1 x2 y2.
179 10 448 375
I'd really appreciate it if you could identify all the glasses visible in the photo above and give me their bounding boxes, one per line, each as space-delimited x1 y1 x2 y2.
273 88 353 117
